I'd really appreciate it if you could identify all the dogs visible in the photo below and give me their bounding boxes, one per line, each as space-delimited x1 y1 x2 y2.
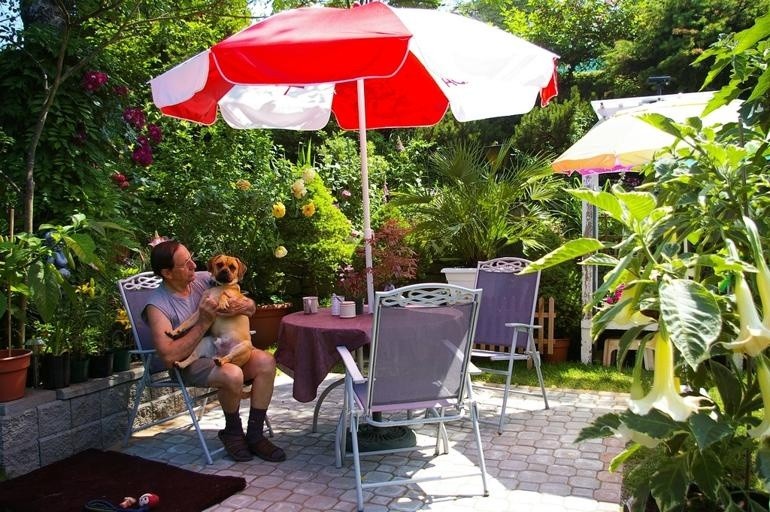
165 255 251 400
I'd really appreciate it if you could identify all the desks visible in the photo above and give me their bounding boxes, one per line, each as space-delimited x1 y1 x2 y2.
273 304 427 457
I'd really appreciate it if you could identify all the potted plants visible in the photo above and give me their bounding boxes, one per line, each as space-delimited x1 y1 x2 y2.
512 10 770 512
0 212 132 403
540 269 582 364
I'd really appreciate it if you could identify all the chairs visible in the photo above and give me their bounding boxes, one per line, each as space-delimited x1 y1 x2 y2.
335 283 489 512
117 269 274 465
469 256 549 435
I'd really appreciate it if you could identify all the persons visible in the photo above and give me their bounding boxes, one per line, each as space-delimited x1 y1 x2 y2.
140 239 290 462
147 0 562 314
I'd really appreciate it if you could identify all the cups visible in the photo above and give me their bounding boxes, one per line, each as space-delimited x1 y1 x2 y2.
303 297 317 314
340 301 356 318
331 296 344 316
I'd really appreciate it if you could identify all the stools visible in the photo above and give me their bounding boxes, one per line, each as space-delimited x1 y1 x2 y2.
602 338 654 371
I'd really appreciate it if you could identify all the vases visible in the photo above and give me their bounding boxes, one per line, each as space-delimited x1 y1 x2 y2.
345 297 362 316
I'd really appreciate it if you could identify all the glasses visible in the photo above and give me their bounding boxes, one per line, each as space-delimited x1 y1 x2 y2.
175 252 194 268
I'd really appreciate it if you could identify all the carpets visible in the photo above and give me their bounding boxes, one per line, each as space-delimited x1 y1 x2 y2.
0 446 246 512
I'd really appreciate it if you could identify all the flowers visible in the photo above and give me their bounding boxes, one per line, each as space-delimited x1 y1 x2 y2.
337 263 375 298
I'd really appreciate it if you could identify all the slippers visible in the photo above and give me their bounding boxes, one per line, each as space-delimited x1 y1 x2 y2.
218 430 286 462
